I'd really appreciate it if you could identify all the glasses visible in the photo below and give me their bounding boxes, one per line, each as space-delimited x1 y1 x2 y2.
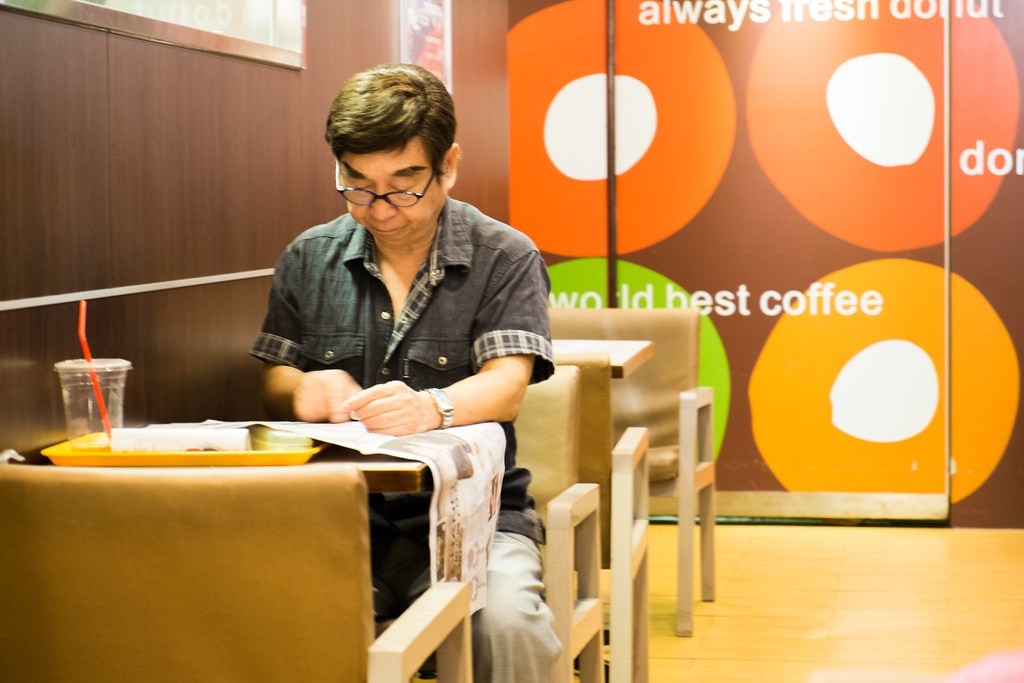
334 156 433 208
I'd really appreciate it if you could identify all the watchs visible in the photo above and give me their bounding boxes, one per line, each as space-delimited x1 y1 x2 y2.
424 385 454 433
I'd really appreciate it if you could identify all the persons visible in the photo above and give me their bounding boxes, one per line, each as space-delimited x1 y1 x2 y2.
247 64 562 683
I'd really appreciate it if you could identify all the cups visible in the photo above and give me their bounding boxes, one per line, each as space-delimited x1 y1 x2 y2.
54 358 132 451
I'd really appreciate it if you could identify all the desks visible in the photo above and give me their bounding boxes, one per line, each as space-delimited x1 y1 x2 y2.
546 341 654 377
39 430 497 493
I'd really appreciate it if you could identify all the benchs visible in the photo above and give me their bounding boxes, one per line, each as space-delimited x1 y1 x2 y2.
0 306 719 683
